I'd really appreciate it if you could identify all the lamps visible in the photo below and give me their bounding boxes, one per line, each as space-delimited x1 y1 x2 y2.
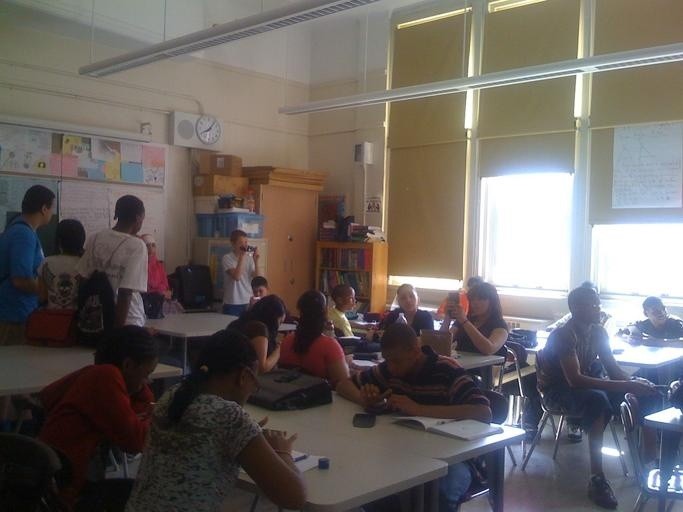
50 1 394 79
268 1 681 117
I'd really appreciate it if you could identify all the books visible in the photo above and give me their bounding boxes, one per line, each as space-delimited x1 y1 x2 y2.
319 220 338 241
354 300 370 313
321 248 372 270
320 270 369 297
347 222 384 241
389 417 503 441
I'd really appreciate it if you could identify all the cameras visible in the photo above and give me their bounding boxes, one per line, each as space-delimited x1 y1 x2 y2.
246 246 254 253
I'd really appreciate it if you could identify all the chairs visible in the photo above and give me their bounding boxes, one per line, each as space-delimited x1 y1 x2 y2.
616 402 683 512
625 392 657 471
492 345 518 466
507 348 538 426
457 389 509 512
522 346 628 475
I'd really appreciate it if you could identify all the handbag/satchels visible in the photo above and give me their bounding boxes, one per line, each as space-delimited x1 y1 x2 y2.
25 307 75 347
251 367 332 412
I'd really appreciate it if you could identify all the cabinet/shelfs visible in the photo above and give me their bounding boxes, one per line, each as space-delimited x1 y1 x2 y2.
242 164 330 311
315 240 387 315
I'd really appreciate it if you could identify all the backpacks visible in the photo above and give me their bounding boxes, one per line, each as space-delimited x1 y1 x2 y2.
63 231 131 346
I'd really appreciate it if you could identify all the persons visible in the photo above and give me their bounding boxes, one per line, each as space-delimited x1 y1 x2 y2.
375 284 434 338
327 284 355 336
76 196 147 327
35 326 159 511
123 329 308 512
337 323 492 512
248 276 269 309
222 231 260 317
523 283 611 443
140 234 172 301
536 287 665 510
615 297 683 346
440 284 508 365
227 293 286 374
280 291 350 389
438 277 487 320
0 186 55 433
37 220 85 310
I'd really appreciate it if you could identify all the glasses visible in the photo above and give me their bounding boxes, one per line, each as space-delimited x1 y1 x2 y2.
239 361 262 394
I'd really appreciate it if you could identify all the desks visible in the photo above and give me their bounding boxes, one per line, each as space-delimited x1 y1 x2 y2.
0 345 183 434
595 345 683 409
144 311 299 373
644 405 683 511
237 391 525 512
345 349 517 464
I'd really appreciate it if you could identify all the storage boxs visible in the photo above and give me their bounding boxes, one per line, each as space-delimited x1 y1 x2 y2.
209 154 242 176
196 215 218 237
195 196 218 213
218 214 264 239
194 174 249 195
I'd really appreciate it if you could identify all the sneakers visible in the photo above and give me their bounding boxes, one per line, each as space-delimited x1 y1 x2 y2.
588 471 618 508
568 425 582 441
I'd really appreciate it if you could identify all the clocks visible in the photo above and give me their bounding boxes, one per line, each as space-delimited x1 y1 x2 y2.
197 115 221 144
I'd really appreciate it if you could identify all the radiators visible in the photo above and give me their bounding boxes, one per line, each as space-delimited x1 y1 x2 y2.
504 317 552 329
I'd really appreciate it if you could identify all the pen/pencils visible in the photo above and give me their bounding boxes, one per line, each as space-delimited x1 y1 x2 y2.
293 453 311 461
437 417 463 424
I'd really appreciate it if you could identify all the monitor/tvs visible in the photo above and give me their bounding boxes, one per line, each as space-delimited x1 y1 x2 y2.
167 264 213 310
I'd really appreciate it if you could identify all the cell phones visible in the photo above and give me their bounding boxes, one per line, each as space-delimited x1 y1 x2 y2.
447 292 461 319
